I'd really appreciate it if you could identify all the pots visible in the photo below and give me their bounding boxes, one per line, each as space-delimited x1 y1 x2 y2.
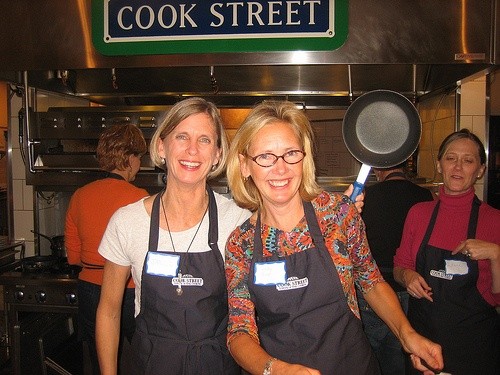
21 256 52 272
31 230 64 247
341 90 422 202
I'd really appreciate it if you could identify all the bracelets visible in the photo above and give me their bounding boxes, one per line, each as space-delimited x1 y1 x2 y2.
262 357 278 375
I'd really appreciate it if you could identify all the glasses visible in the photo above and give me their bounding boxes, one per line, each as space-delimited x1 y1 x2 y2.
242 149 306 167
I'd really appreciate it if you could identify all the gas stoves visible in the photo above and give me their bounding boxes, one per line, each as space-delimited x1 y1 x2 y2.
0 255 83 313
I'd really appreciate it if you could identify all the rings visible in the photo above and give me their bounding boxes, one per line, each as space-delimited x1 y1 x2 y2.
410 292 415 295
465 250 471 257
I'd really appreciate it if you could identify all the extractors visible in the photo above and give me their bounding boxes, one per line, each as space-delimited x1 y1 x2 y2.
26 108 167 192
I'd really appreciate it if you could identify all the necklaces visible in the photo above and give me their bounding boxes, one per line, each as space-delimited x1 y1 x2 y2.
160 196 209 295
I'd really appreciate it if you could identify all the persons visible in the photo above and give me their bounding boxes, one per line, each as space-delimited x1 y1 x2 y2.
393 127 500 375
224 99 444 375
356 162 433 375
64 120 149 375
96 97 365 375
